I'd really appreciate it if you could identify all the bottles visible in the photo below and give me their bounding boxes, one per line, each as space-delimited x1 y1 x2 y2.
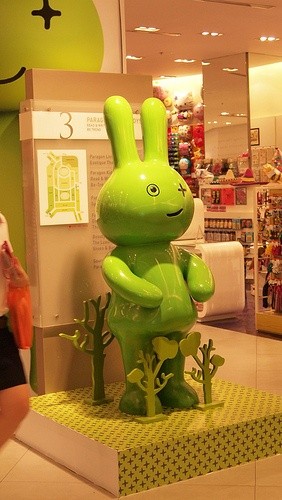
1 247 29 289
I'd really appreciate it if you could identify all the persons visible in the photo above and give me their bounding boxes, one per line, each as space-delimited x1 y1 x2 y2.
0 208 31 449
